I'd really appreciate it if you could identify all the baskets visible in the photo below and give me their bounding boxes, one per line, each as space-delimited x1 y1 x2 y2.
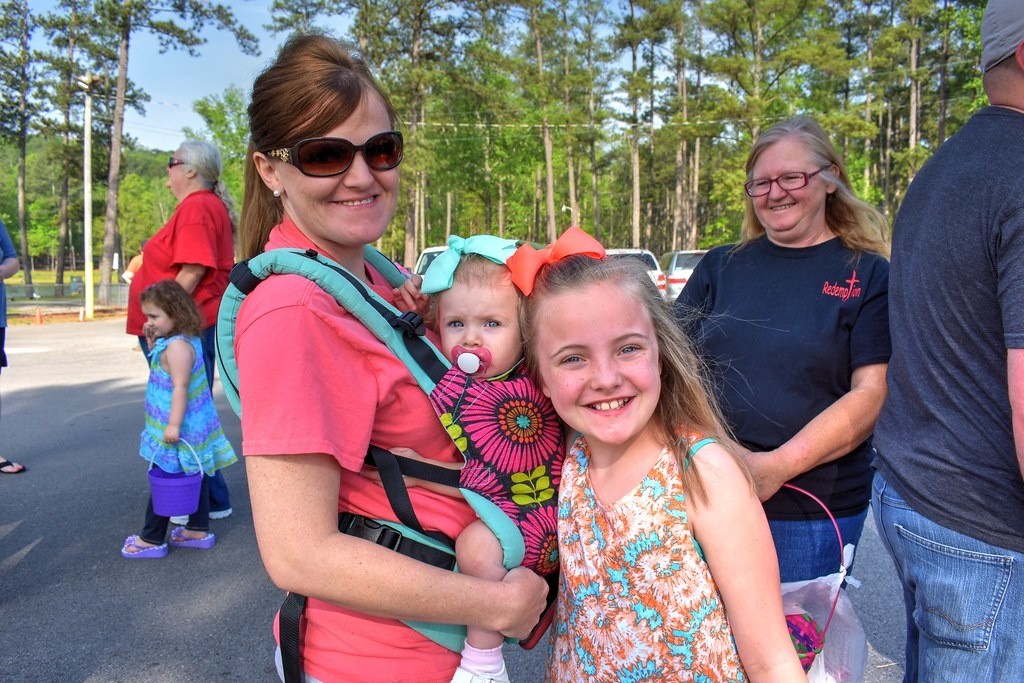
782 482 846 675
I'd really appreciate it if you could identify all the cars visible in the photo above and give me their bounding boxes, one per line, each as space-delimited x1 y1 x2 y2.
660 249 711 301
605 248 667 300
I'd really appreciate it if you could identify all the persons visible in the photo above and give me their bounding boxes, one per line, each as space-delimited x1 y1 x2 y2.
871 0 1023 683
121 279 238 558
235 29 550 683
126 138 236 523
670 116 891 585
393 234 569 683
506 226 810 683
0 221 25 475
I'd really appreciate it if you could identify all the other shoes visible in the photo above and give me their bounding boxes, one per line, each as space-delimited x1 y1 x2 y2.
450 659 510 683
169 506 232 526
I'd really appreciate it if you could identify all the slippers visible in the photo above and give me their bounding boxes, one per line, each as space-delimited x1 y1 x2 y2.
168 527 215 549
121 535 169 559
0 460 26 474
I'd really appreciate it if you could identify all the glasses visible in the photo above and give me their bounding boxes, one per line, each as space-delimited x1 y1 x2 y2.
263 130 404 178
169 157 183 169
744 166 830 197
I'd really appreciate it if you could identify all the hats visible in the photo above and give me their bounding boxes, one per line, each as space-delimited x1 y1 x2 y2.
981 0 1024 72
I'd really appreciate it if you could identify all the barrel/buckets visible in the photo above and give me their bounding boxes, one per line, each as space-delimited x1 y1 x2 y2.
148 467 204 518
